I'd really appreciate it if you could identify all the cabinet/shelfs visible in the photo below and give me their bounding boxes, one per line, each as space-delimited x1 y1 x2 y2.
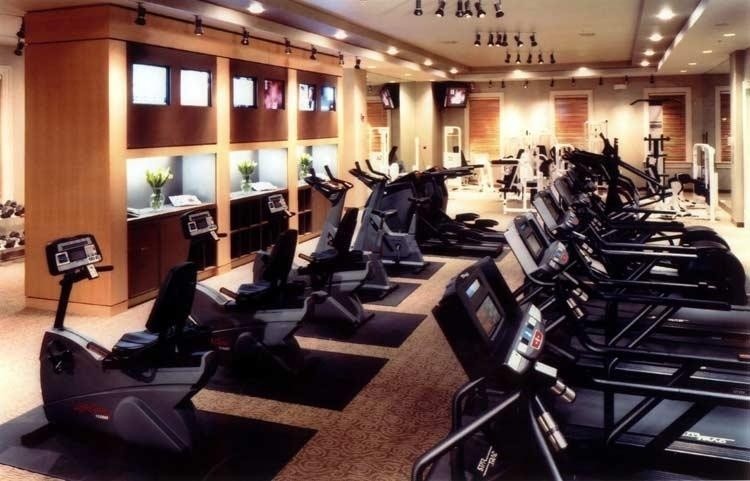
291 45 344 244
26 42 222 314
224 33 295 265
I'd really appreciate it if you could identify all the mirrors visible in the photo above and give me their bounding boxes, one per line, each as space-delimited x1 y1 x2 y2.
644 86 692 163
716 86 733 165
463 91 505 184
549 89 592 150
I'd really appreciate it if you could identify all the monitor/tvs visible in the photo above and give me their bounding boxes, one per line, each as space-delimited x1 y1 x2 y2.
263 79 285 111
441 81 472 109
319 85 336 112
298 83 317 112
233 75 259 109
180 68 212 108
380 83 399 110
132 61 172 107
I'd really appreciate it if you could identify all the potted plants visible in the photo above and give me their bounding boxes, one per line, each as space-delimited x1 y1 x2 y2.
297 152 314 182
144 166 174 211
237 159 259 193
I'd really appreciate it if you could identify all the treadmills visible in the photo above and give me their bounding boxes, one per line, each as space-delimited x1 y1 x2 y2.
529 191 750 339
505 210 750 396
410 383 750 481
428 255 750 466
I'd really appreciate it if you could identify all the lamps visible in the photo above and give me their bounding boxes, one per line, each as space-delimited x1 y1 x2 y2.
504 52 556 64
413 0 505 20
475 29 538 48
470 74 658 89
